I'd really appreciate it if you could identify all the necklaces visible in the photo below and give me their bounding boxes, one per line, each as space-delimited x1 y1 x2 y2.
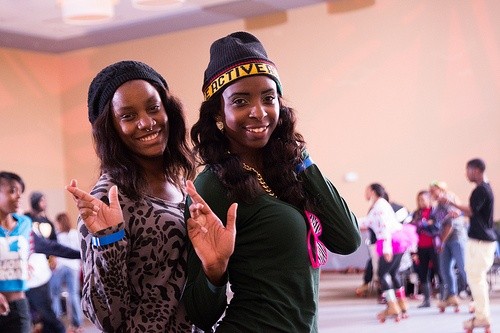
242 163 278 199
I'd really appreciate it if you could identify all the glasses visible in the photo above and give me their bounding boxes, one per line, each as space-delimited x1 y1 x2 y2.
304 211 328 269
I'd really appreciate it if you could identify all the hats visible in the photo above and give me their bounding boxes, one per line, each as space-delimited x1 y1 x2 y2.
200 31 284 101
87 59 171 124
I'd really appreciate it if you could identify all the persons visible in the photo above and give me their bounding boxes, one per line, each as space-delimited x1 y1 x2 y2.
355 182 475 323
0 172 82 333
66 60 203 333
185 32 360 333
448 159 498 333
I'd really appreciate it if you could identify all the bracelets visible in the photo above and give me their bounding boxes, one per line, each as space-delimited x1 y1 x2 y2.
91 229 125 246
295 157 314 174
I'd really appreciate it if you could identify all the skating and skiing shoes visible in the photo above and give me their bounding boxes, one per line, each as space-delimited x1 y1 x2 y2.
398 298 408 319
463 316 491 333
438 297 460 312
377 300 401 323
